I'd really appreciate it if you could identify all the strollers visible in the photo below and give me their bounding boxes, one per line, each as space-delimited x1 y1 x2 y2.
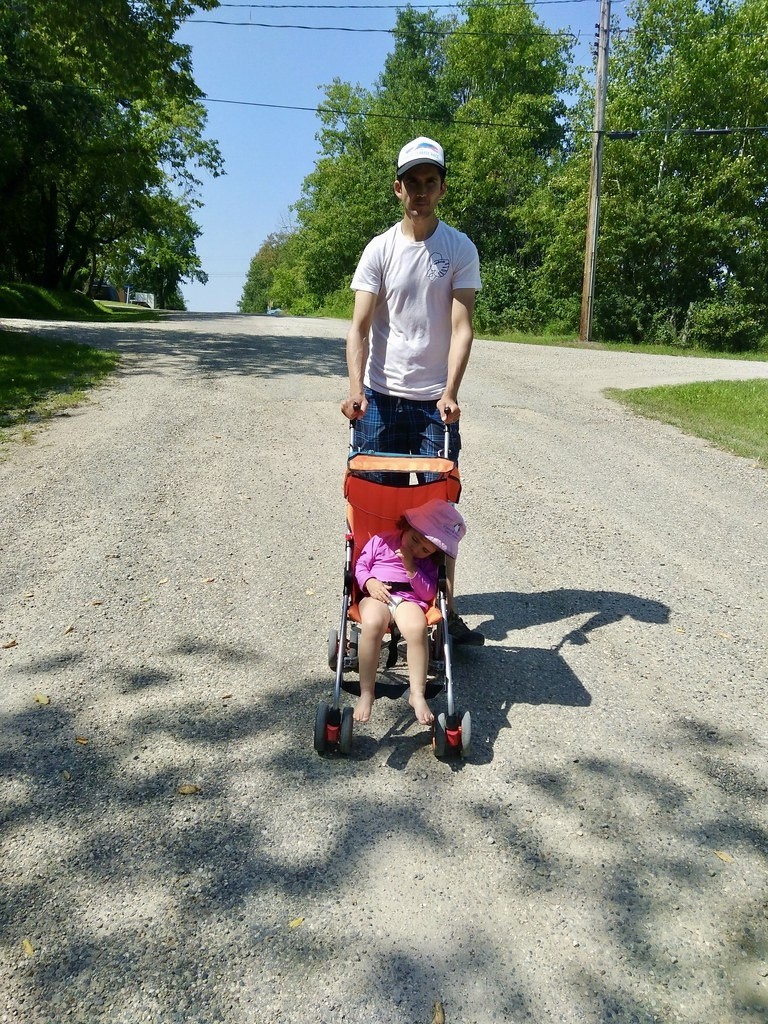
313 400 473 758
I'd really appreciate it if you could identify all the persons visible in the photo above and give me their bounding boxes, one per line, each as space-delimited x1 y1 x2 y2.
341 136 486 643
352 498 467 725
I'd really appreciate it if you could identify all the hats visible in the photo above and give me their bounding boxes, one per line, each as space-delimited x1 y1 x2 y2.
397 136 446 176
403 498 466 560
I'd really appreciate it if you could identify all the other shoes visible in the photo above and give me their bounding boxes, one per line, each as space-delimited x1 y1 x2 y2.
448 609 485 646
